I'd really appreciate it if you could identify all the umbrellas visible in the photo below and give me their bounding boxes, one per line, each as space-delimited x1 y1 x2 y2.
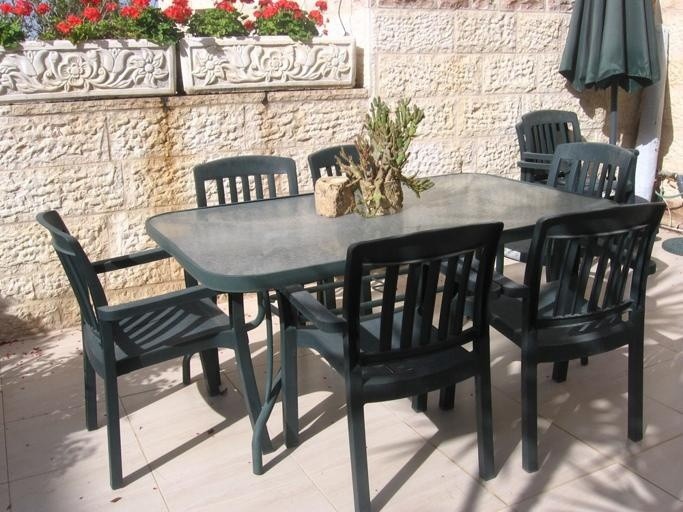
557 0 662 177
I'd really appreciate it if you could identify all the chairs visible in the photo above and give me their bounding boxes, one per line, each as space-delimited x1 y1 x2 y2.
145 145 663 510
36 208 275 490
514 109 598 190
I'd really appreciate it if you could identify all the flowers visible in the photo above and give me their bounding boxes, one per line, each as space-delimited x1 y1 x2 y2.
0 1 333 48
